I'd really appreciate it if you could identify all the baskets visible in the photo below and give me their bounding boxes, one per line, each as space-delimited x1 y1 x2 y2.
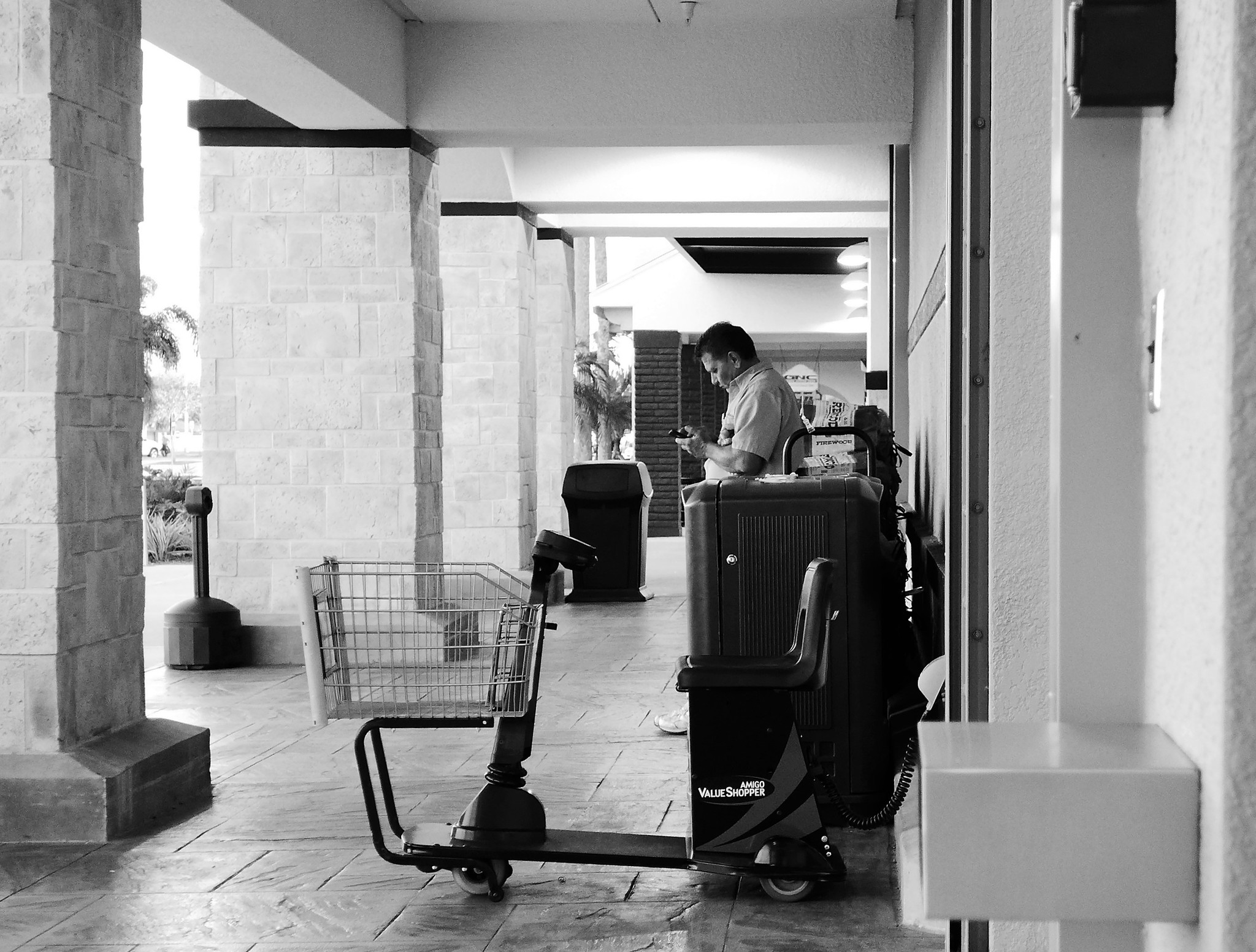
292 555 544 727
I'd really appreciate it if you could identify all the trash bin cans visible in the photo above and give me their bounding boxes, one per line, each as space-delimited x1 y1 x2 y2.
562 459 656 601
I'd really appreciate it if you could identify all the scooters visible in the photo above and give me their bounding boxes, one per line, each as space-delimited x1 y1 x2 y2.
294 528 849 901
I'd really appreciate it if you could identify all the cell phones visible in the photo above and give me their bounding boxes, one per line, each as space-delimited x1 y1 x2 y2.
669 429 690 438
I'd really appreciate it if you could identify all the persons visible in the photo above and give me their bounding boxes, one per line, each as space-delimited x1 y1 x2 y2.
648 320 803 738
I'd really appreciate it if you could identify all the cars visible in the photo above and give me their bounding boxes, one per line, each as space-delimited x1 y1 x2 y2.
141 436 160 459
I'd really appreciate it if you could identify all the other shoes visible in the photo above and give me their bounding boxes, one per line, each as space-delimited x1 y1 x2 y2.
653 702 688 735
